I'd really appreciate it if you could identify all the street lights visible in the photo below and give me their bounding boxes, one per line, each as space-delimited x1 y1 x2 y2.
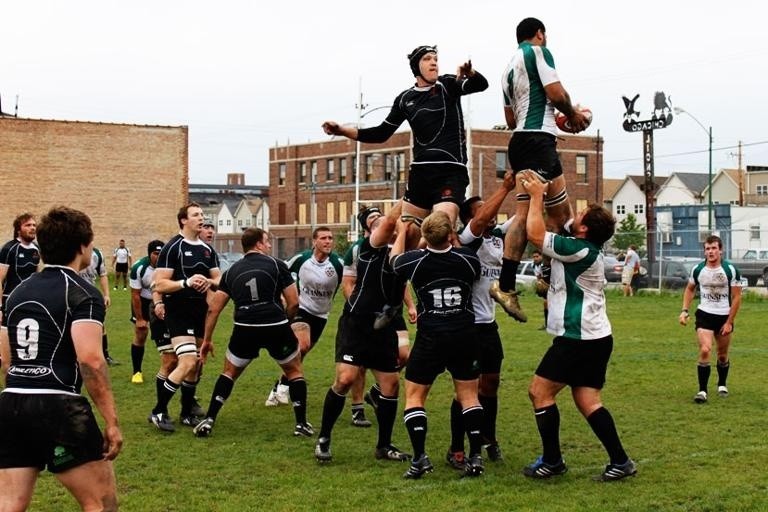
673 107 713 236
328 103 392 265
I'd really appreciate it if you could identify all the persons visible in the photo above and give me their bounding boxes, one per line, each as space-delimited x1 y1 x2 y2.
323 41 487 256
316 169 641 487
488 13 592 323
111 239 133 293
1 214 44 322
75 245 117 366
128 201 343 437
1 204 126 511
679 235 744 410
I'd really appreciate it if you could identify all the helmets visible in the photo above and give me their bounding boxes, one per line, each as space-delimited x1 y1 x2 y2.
360 208 381 227
410 46 437 74
148 241 164 256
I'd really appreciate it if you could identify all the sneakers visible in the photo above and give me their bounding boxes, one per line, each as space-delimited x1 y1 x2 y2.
489 280 527 322
374 305 401 329
694 391 707 403
718 386 728 396
532 280 550 299
107 356 122 365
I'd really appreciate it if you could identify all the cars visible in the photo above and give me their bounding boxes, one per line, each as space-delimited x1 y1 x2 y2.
512 260 538 285
602 255 766 288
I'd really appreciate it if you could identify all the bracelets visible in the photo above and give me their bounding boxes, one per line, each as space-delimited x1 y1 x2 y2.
680 309 689 313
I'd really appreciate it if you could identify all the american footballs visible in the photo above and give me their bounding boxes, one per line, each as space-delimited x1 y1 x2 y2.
556 102 594 133
